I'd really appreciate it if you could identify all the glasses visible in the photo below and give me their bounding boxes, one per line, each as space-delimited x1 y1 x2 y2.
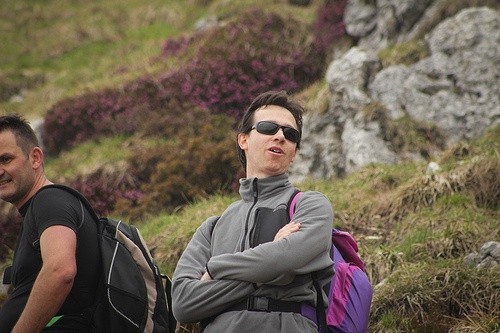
243 120 301 147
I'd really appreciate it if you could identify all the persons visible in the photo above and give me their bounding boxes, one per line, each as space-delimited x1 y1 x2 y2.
170 90 333 333
0 114 102 333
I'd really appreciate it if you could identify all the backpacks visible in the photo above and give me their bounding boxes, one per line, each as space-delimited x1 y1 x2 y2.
25 184 175 333
286 189 373 333
